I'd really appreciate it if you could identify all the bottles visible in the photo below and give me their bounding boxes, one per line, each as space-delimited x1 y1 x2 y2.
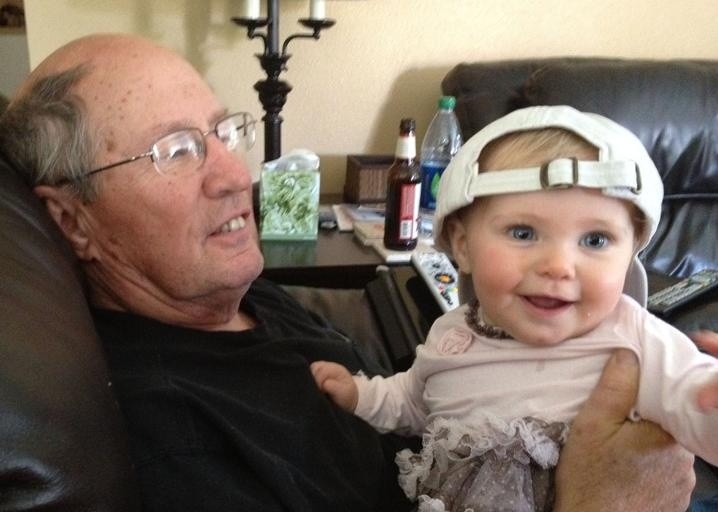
384 96 462 252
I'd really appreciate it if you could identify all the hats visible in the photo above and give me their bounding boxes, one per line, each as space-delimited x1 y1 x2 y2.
432 105 665 310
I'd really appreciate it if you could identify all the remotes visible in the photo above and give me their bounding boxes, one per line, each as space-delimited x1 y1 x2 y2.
647 268 718 318
409 249 459 316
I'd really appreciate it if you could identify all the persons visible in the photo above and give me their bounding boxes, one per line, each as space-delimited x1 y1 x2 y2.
312 106 718 512
1 31 697 512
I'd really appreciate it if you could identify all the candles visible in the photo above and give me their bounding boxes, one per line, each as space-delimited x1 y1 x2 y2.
239 1 261 20
309 1 327 20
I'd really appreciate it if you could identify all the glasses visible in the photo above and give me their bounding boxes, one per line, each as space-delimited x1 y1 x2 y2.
85 112 258 179
